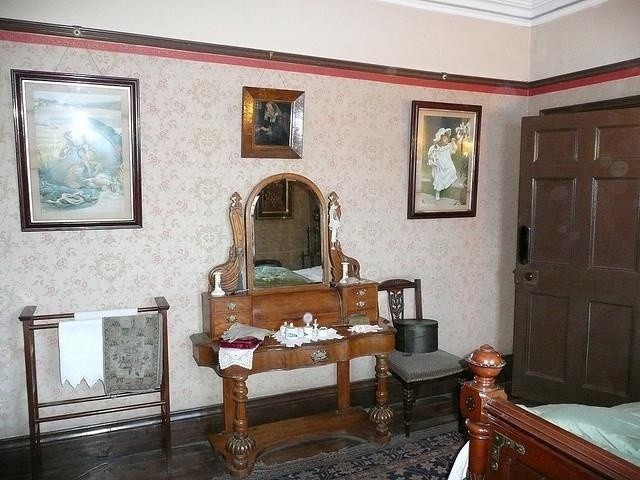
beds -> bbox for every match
[445,345,640,478]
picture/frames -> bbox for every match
[241,87,305,159]
[407,99,482,219]
[10,68,143,231]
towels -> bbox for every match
[57,306,164,398]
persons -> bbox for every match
[254,102,288,145]
[426,127,458,200]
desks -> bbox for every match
[191,276,398,477]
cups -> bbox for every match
[280,321,299,339]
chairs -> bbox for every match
[376,278,469,437]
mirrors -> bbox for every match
[245,173,330,295]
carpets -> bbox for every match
[211,419,469,480]
